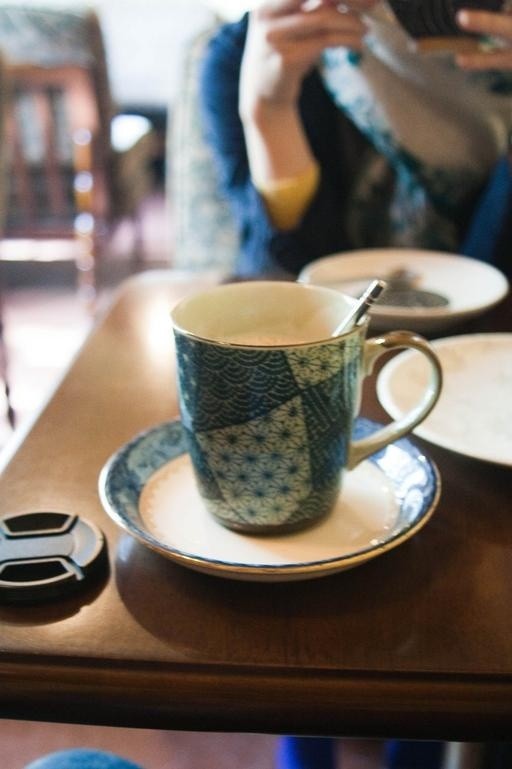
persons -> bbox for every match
[198,1,511,306]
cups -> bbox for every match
[170,279,443,538]
[346,0,508,53]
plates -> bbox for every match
[297,248,510,329]
[97,414,442,582]
[375,332,511,469]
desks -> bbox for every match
[0,264,511,767]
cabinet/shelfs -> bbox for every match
[2,0,109,295]
